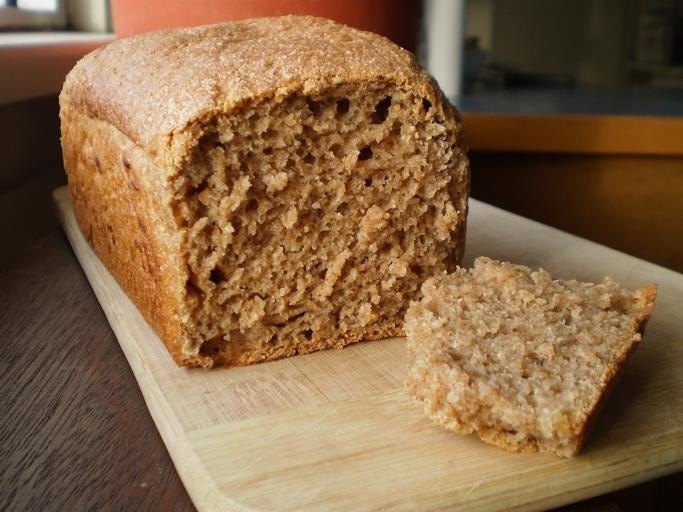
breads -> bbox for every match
[400,255,657,457]
[58,14,469,366]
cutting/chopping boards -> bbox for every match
[50,177,681,510]
